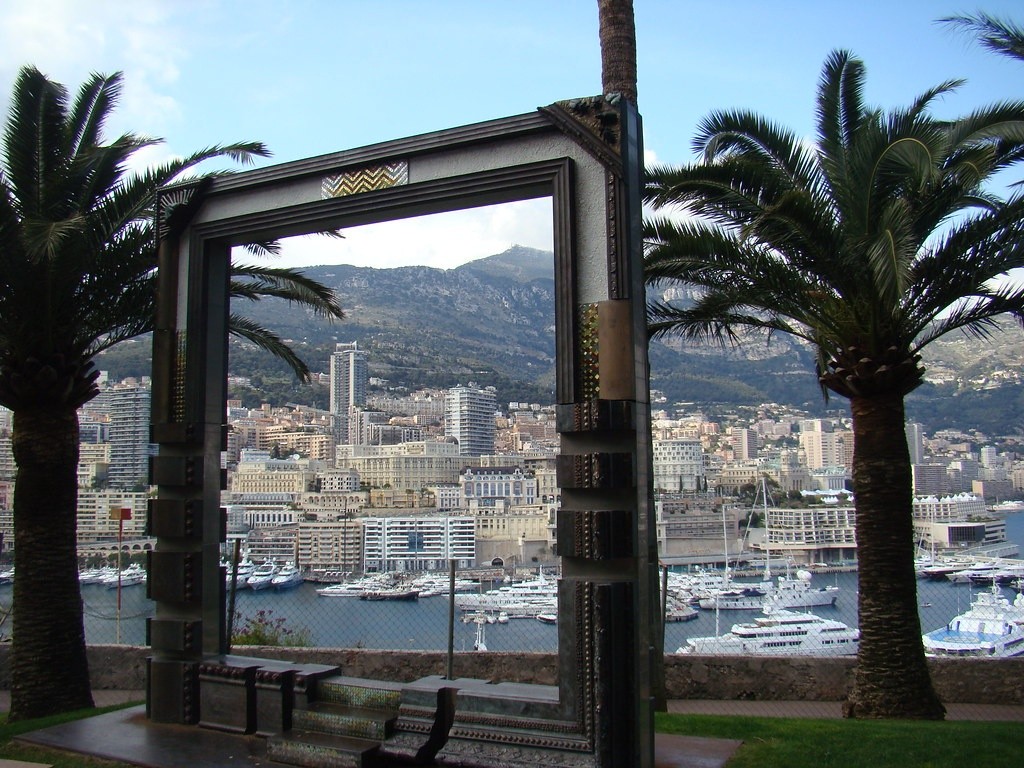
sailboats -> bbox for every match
[701,478,840,609]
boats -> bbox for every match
[675,609,859,656]
[1,562,308,592]
[917,550,1024,660]
[314,569,484,600]
[461,610,556,624]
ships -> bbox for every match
[442,574,698,622]
